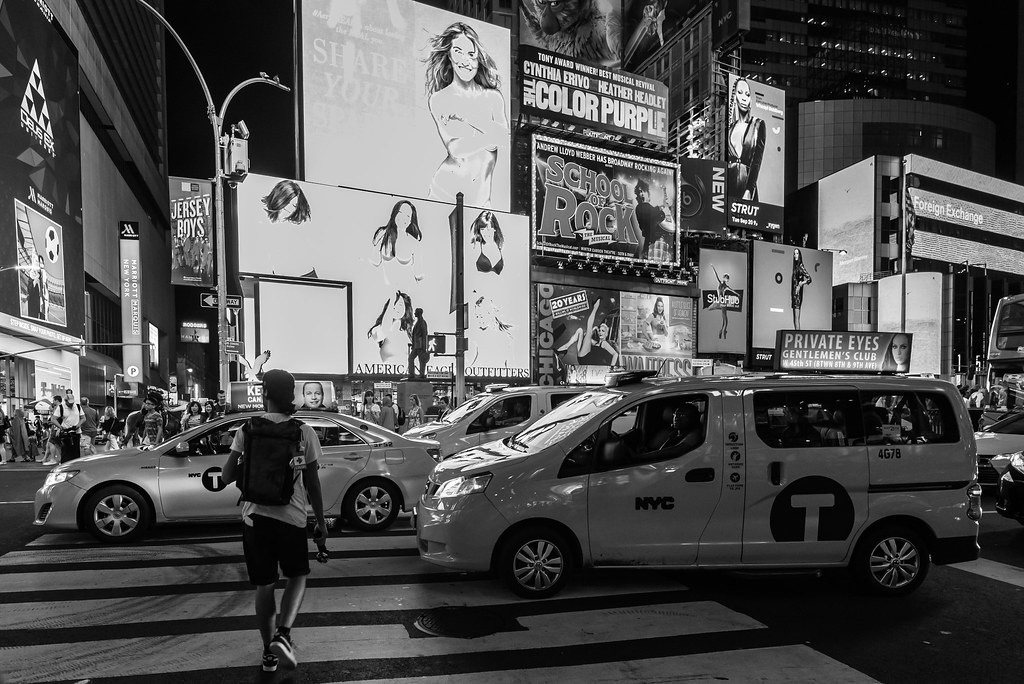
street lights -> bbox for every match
[113,372,126,418]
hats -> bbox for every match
[144,397,159,404]
[255,369,295,397]
[65,394,75,404]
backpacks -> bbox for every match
[392,403,406,425]
[234,416,305,507]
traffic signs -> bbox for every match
[200,291,247,313]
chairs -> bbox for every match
[644,403,680,452]
[813,407,848,447]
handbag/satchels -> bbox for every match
[49,424,63,444]
[3,416,11,430]
[101,431,111,443]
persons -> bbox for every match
[781,402,822,449]
[791,248,813,331]
[709,265,740,339]
[259,21,676,384]
[27,255,51,323]
[501,398,529,425]
[881,334,912,372]
[406,307,430,379]
[0,389,230,467]
[625,402,704,460]
[220,369,328,672]
[300,382,326,408]
[727,77,766,202]
[360,391,451,432]
[172,234,213,277]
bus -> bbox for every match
[984,295,1024,404]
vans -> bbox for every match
[411,331,985,601]
[402,382,607,462]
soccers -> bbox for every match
[45,225,60,263]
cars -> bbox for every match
[962,407,1024,525]
[32,409,444,547]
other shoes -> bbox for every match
[43,461,59,465]
[261,650,279,671]
[22,457,30,462]
[270,632,298,670]
[6,459,16,462]
